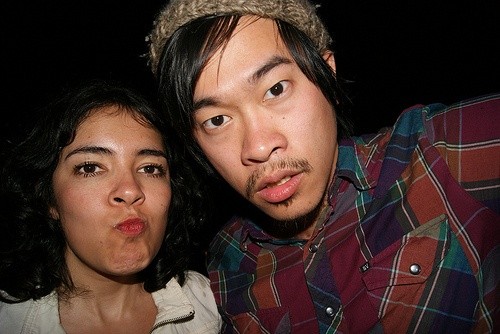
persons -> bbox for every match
[0,79,223,334]
[142,0,500,332]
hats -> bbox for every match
[145,0,331,73]
